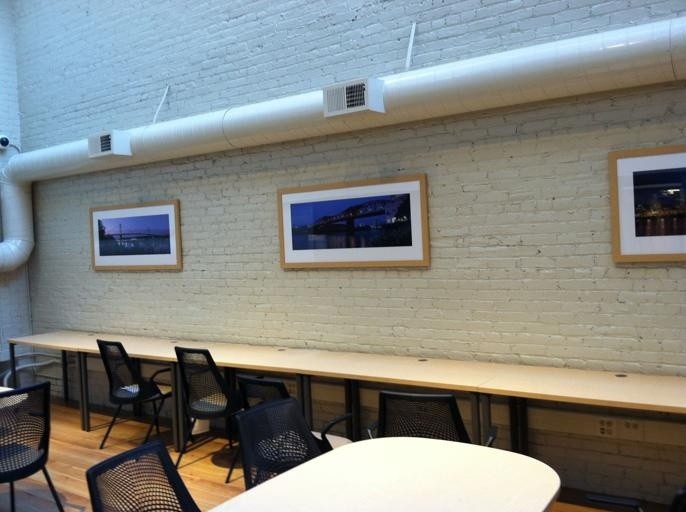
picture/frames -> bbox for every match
[277,173,431,270]
[607,144,686,264]
[88,200,184,272]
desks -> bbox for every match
[303,351,500,445]
[7,330,99,429]
[201,436,562,511]
[78,335,198,453]
[178,347,328,453]
[0,386,14,441]
[477,364,686,450]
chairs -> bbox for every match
[173,346,265,484]
[98,338,174,461]
[234,396,321,492]
[236,373,327,455]
[367,392,496,449]
[85,441,203,512]
[0,379,66,512]
